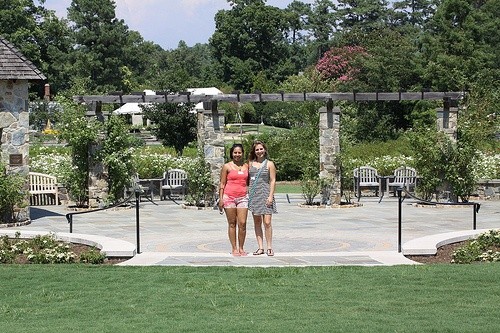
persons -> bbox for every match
[218,144,249,256]
[247,140,278,256]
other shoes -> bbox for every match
[238,251,247,256]
[232,251,242,256]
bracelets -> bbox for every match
[219,188,224,191]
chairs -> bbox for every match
[386,166,417,198]
[354,165,383,203]
[162,170,188,201]
[125,172,154,203]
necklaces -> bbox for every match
[238,168,241,171]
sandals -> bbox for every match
[253,249,264,255]
[267,249,275,256]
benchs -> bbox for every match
[29,172,59,206]
